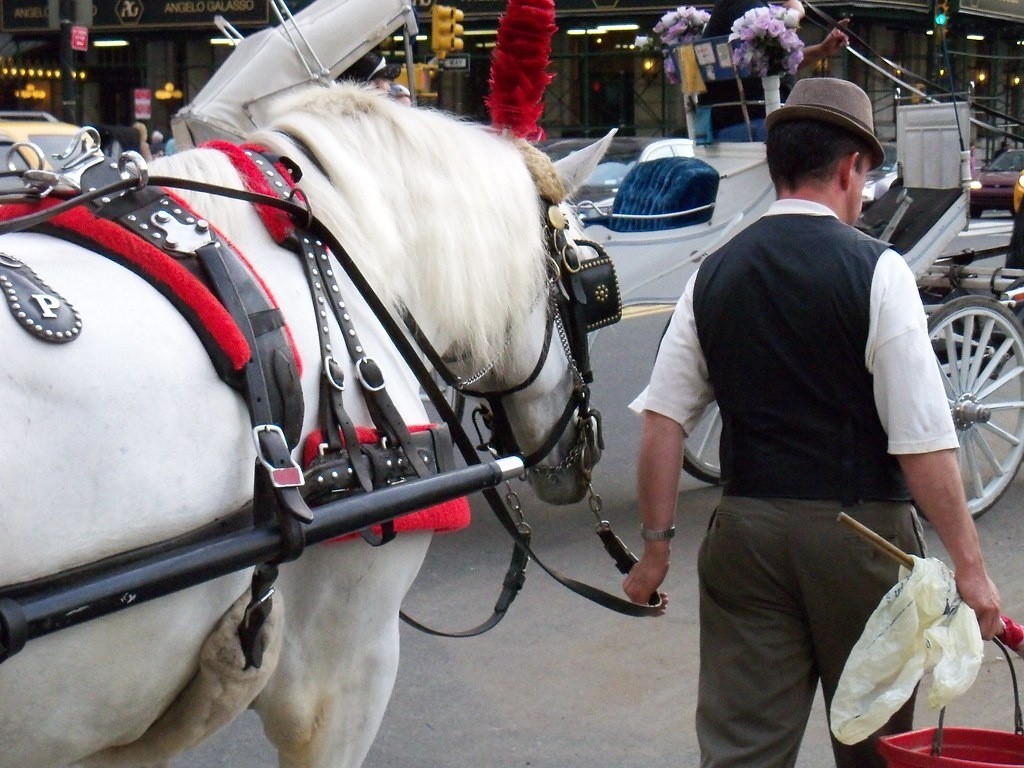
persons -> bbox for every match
[131,121,178,162]
[701,0,849,120]
[340,52,411,106]
[622,77,1001,768]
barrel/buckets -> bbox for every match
[878,633,1024,768]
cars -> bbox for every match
[541,136,671,217]
[970,149,1024,218]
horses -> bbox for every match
[0,82,618,768]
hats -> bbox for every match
[763,77,885,171]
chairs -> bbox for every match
[579,157,719,272]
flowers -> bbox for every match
[730,6,804,78]
[652,7,710,48]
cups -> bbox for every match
[75,0,97,26]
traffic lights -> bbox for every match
[934,13,948,28]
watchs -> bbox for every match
[640,524,676,541]
